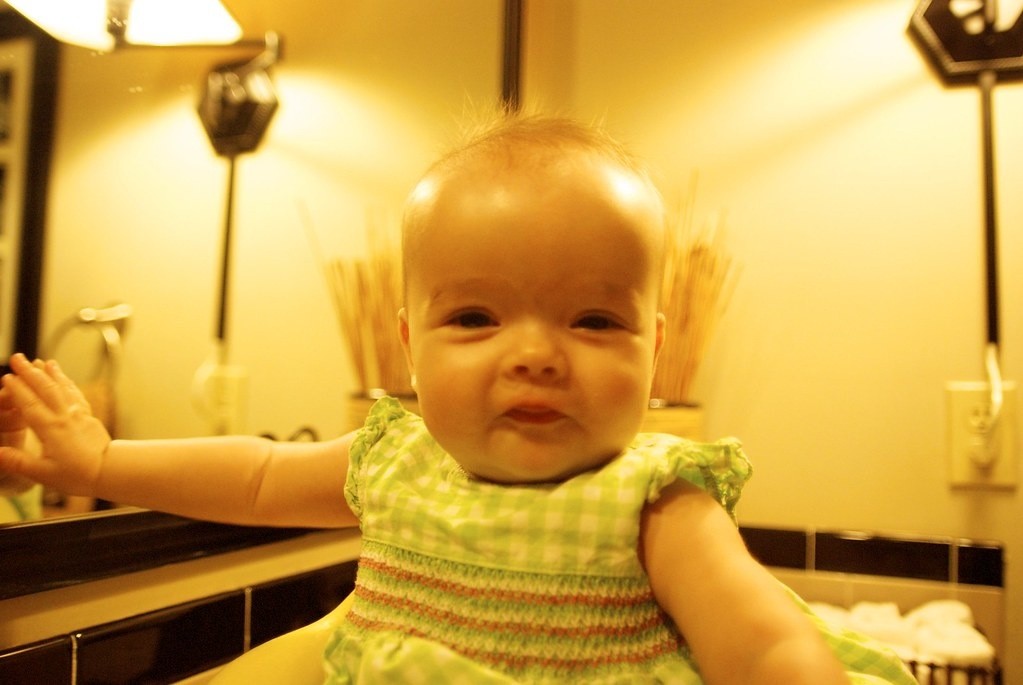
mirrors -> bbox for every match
[0,0,524,598]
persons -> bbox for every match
[0,117,919,685]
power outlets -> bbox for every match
[952,381,1016,485]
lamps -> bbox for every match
[3,0,243,52]
